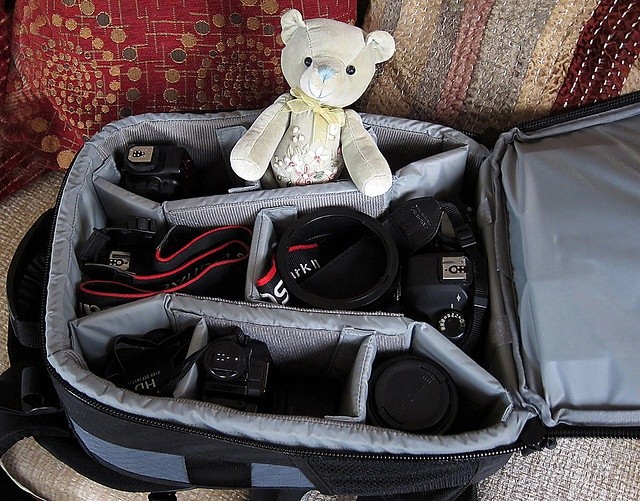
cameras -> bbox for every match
[276,207,474,341]
[120,144,191,199]
[196,340,270,410]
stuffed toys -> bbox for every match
[231,10,395,199]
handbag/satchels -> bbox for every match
[0,90,640,500]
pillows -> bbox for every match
[355,1,639,144]
[1,0,359,169]
